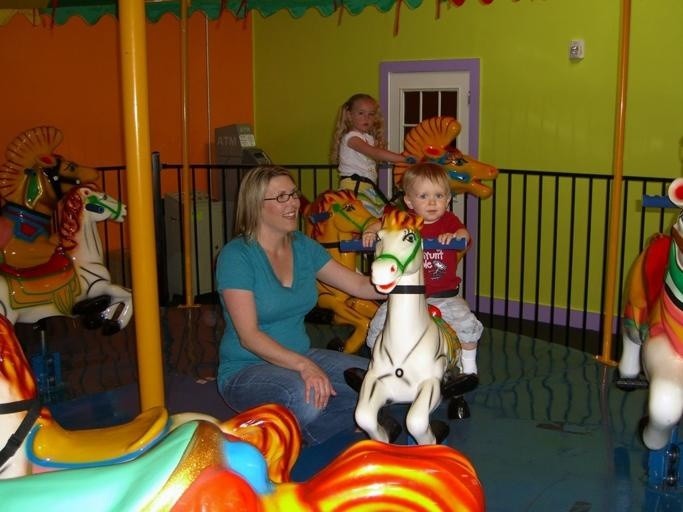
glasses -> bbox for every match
[263,189,301,203]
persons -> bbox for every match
[331,94,408,218]
[362,164,484,377]
[216,165,388,450]
